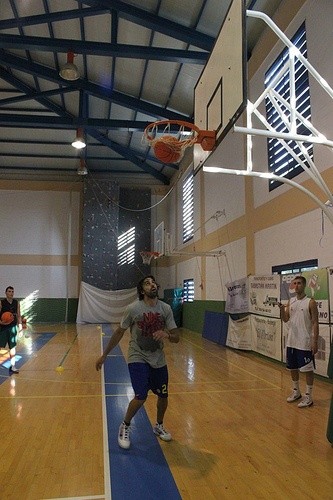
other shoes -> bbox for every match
[9,367,19,373]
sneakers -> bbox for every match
[118,421,130,449]
[153,423,172,441]
[287,394,301,402]
[298,394,312,407]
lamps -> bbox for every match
[71,136,86,149]
[59,52,80,81]
[77,160,88,175]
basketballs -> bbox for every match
[154,136,180,163]
[1,312,13,322]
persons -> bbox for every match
[277,276,318,407]
[96,275,180,450]
[0,285,21,373]
[326,342,333,447]
